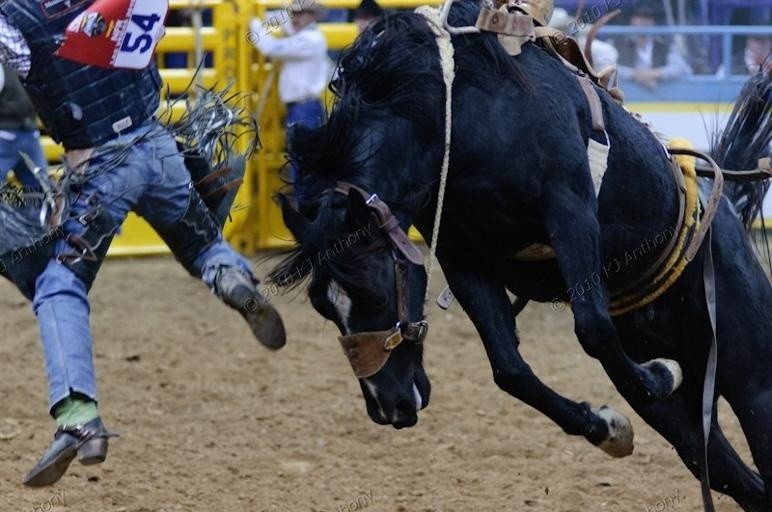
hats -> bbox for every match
[285,1,326,21]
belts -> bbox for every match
[288,97,319,107]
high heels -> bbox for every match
[22,396,110,487]
[213,264,287,351]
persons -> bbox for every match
[711,22,772,75]
[352,1,385,32]
[551,8,618,86]
[248,1,335,198]
[603,4,686,91]
[0,0,289,487]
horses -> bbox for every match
[250,10,771,511]
